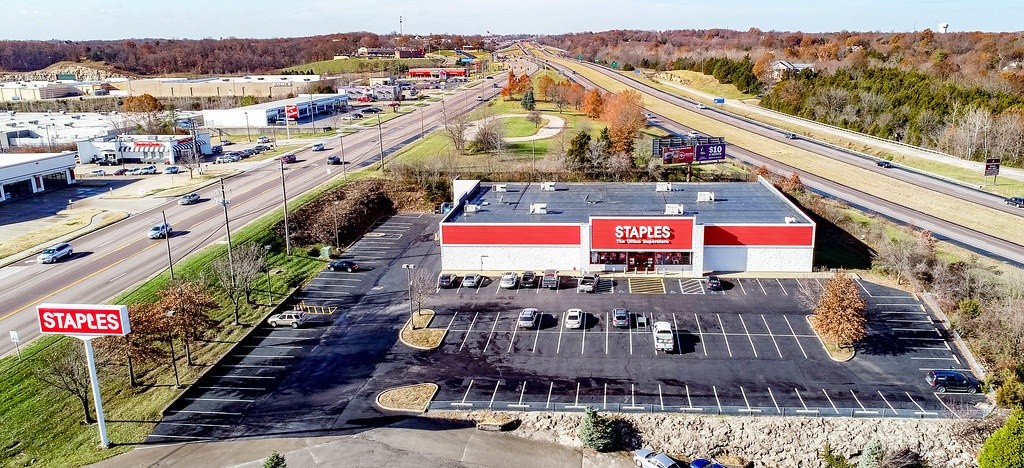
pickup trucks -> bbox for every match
[578,272,601,292]
[520,270,537,288]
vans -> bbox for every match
[652,321,675,354]
[540,269,561,289]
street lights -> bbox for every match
[401,262,417,331]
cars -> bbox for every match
[646,113,653,119]
[688,129,700,138]
[313,143,325,151]
[178,193,201,204]
[925,368,982,395]
[611,307,629,327]
[90,164,180,175]
[327,156,342,165]
[565,308,583,329]
[783,132,797,140]
[499,271,519,288]
[633,449,681,468]
[213,136,274,164]
[438,273,456,288]
[697,103,706,109]
[876,160,891,168]
[517,307,539,328]
[461,273,481,288]
[688,458,725,468]
[37,243,74,265]
[148,222,172,239]
[328,261,361,273]
[341,107,383,119]
[388,101,400,106]
[1004,195,1024,208]
[281,154,296,164]
[703,273,723,291]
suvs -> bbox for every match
[267,311,308,327]
